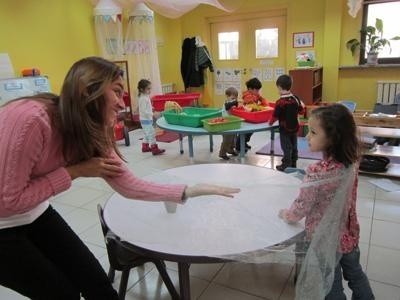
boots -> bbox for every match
[151,144,166,155]
[142,143,152,152]
[292,158,296,168]
[276,159,292,171]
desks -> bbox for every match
[104,163,305,296]
[155,108,280,161]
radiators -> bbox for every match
[376,81,400,114]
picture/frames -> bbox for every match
[291,30,314,49]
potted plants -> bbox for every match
[345,18,399,64]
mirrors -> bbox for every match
[110,59,134,125]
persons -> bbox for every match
[236,78,263,153]
[219,86,238,160]
[268,74,305,171]
[0,57,240,300]
[278,104,376,300]
[137,79,166,157]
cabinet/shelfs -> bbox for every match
[288,66,326,106]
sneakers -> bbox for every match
[245,142,251,148]
[219,153,231,160]
[228,150,239,156]
[236,147,248,153]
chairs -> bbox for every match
[96,204,178,296]
